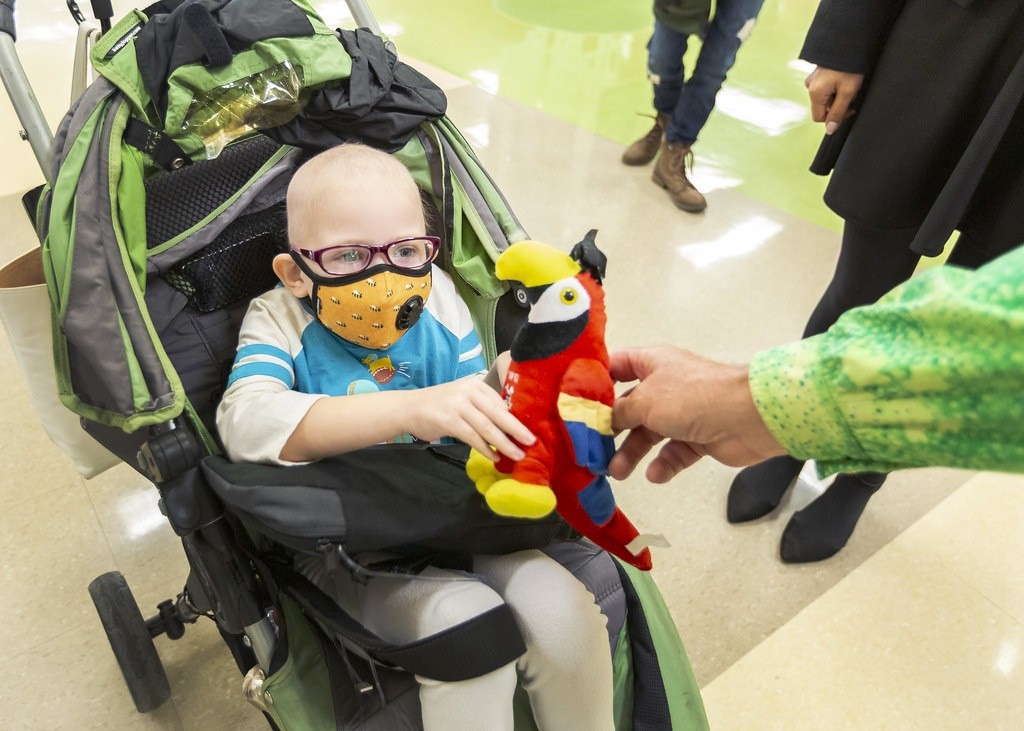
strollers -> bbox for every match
[1,1,713,731]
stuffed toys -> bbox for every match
[466,241,658,573]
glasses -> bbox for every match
[288,235,441,276]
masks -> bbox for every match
[288,250,432,351]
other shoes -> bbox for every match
[727,485,775,524]
[779,511,842,563]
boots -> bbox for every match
[651,138,707,212]
[620,113,672,165]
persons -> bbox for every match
[622,0,766,213]
[725,0,1023,569]
[213,143,618,731]
[603,238,1023,486]
